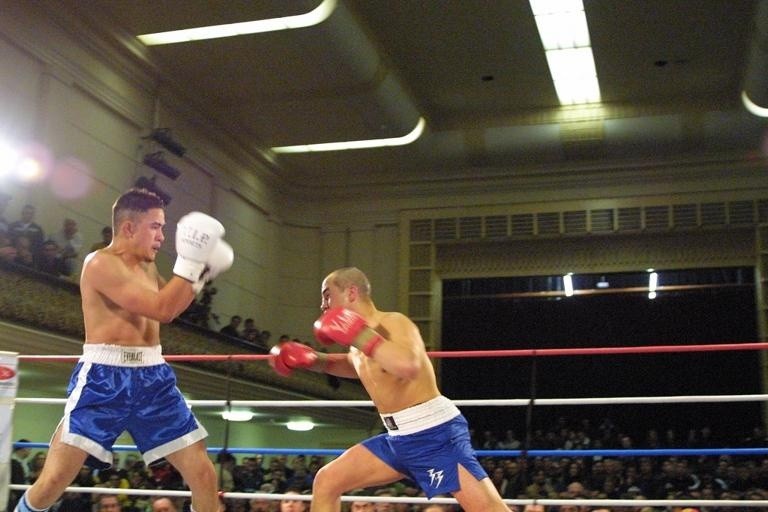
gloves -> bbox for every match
[268,340,328,379]
[312,304,383,357]
[172,211,234,294]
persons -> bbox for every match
[213,449,465,512]
[6,439,192,512]
[2,205,113,284]
[264,264,512,510]
[179,280,328,353]
[12,188,238,511]
[469,415,766,510]
